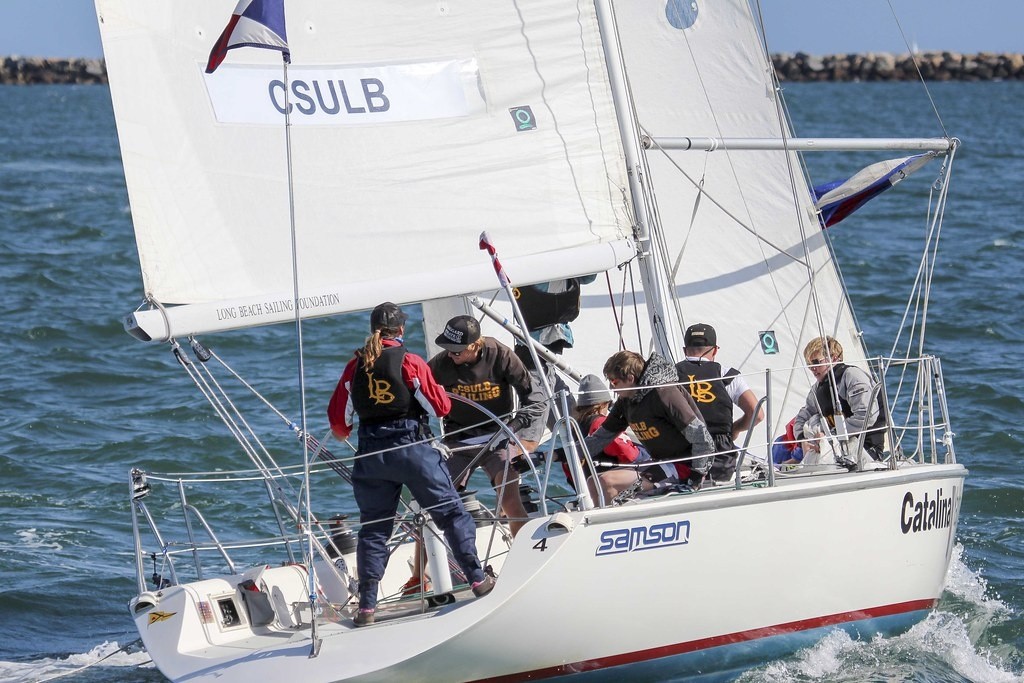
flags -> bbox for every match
[205,0,290,74]
[810,151,933,232]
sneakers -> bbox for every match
[469,571,499,597]
[352,608,375,626]
[399,576,430,594]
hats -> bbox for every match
[577,374,613,406]
[684,323,716,346]
[370,302,409,332]
[435,315,480,353]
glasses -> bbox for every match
[806,357,825,370]
[451,352,460,356]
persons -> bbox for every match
[402,315,546,595]
[327,302,498,627]
[793,335,887,460]
[513,273,598,454]
[561,374,639,493]
[673,323,765,482]
[510,351,715,508]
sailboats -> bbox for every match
[91,0,968,683]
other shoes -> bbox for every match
[523,501,538,513]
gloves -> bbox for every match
[510,452,546,475]
[678,470,706,494]
